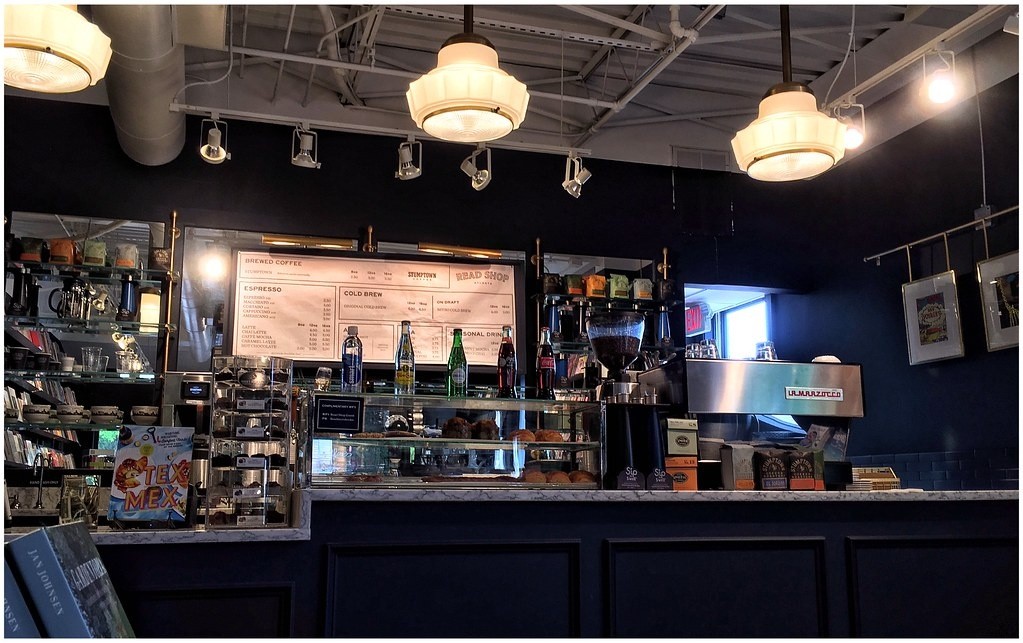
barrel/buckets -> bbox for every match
[613,383,640,404]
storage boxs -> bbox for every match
[719,444,827,491]
[663,417,699,490]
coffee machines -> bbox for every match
[583,304,648,401]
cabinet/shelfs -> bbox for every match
[306,391,606,489]
[531,238,679,400]
[4,207,295,531]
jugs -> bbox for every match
[49,279,91,327]
[189,459,209,489]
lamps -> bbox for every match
[923,49,955,101]
[292,121,321,169]
[394,5,530,190]
[200,114,231,164]
[4,5,114,94]
[685,302,715,338]
[730,5,865,182]
[562,150,591,199]
[1003,11,1019,36]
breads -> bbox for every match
[441,418,500,440]
[503,428,595,484]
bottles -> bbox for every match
[497,326,518,399]
[389,469,398,488]
[555,353,568,389]
[603,302,671,346]
[535,327,552,400]
[395,321,415,399]
[10,495,21,509]
[586,363,598,390]
[448,329,468,400]
[341,326,363,394]
[546,298,595,343]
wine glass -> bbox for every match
[312,366,333,394]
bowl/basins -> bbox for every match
[131,406,159,425]
[57,405,124,425]
[4,405,59,424]
[390,459,400,463]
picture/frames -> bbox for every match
[902,270,964,365]
[977,249,1019,352]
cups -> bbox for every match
[91,356,109,382]
[606,393,657,405]
[61,357,74,371]
[81,347,102,371]
[73,365,81,377]
[685,339,718,359]
[743,342,778,361]
[116,351,144,373]
